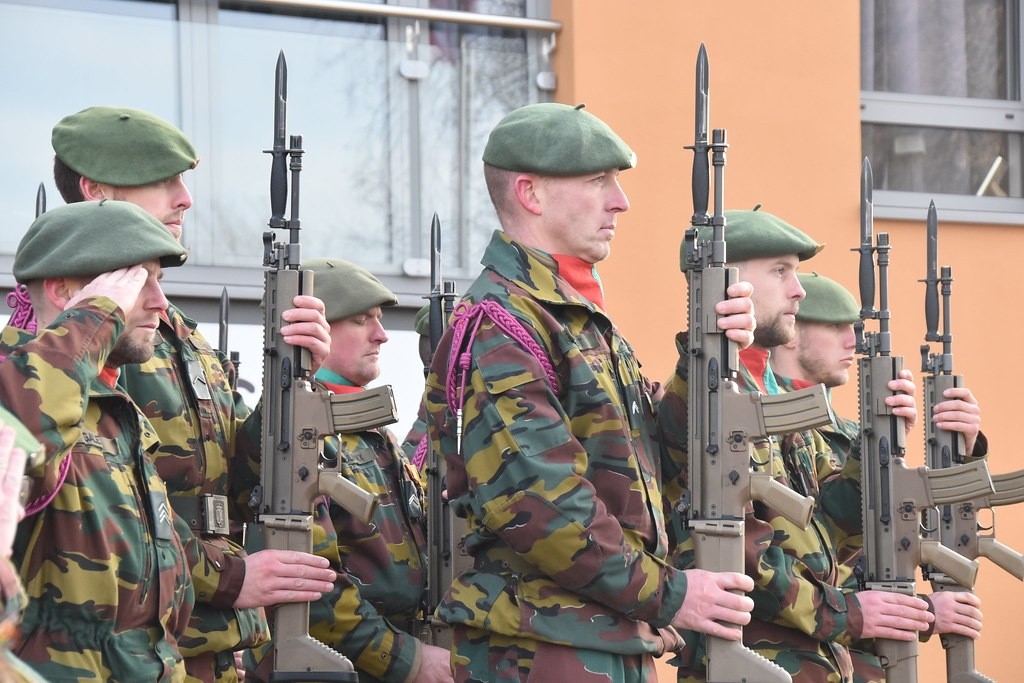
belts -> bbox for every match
[169,494,248,537]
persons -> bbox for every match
[234,254,455,683]
[0,100,342,683]
[402,103,755,683]
[656,209,990,683]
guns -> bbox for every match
[851,155,998,683]
[678,42,835,683]
[416,212,461,653]
[922,198,1024,683]
[217,286,243,389]
[249,46,400,682]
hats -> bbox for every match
[679,203,825,273]
[299,257,399,323]
[414,299,462,337]
[483,102,638,176]
[798,271,864,324]
[51,106,200,186]
[12,198,191,283]
